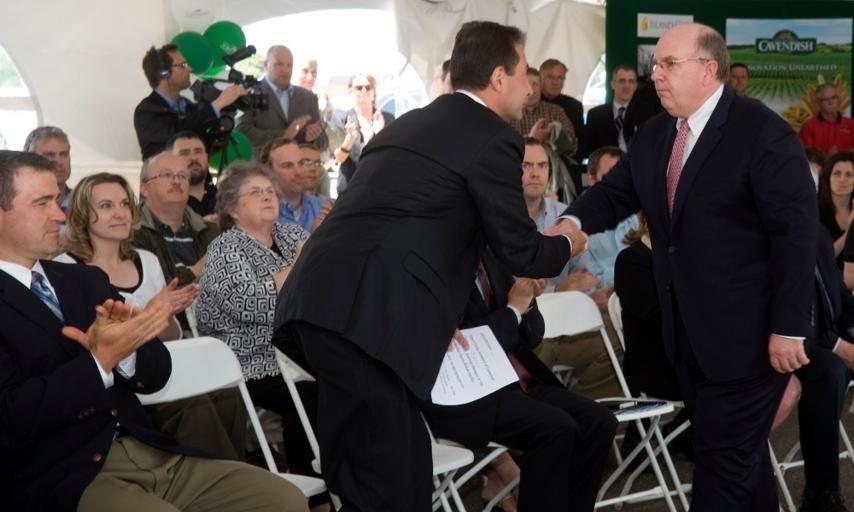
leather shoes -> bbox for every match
[800,486,848,512]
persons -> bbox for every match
[511,57,664,399]
[330,68,397,196]
[270,21,587,512]
[0,128,336,512]
[134,44,249,161]
[771,82,854,512]
[730,62,750,94]
[231,44,335,161]
[419,245,618,511]
[544,24,820,510]
[613,213,803,510]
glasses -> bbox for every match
[144,169,189,184]
[651,56,710,72]
[355,85,372,90]
[817,95,838,102]
[170,61,188,70]
[302,158,325,167]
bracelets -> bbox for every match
[339,146,353,155]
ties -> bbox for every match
[476,260,533,396]
[666,118,689,222]
[30,271,65,327]
[614,107,625,138]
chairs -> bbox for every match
[275,346,475,511]
[765,417,853,512]
[180,285,202,339]
[595,293,695,512]
[132,337,328,511]
[534,292,679,510]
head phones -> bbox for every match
[156,50,171,78]
